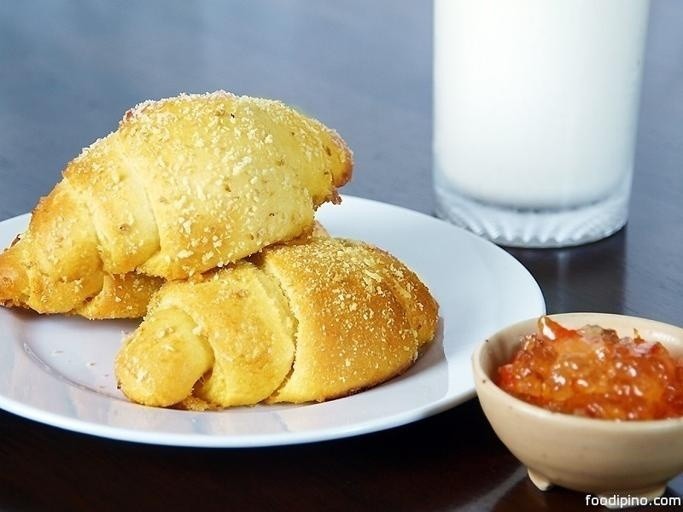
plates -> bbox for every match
[0,195,546,448]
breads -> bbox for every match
[20,93,354,284]
[1,220,331,321]
[116,237,441,413]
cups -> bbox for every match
[433,0,651,249]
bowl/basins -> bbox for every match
[473,312,683,510]
